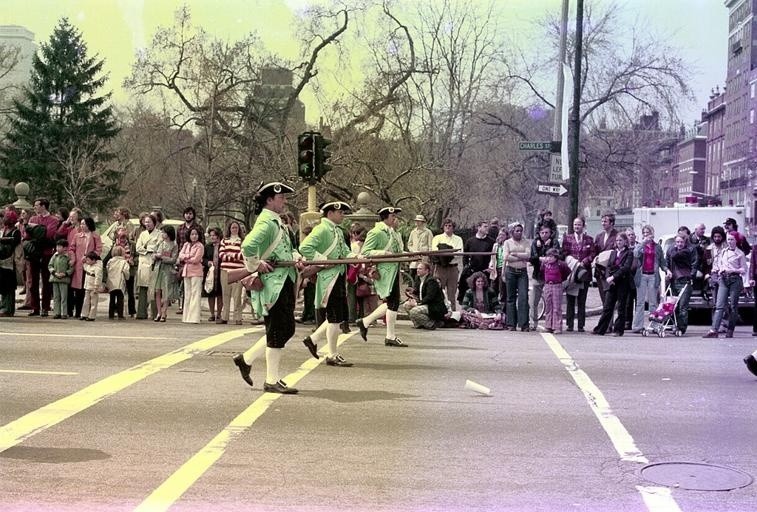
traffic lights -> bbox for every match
[312,133,334,181]
[297,131,315,181]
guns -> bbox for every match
[226,256,421,285]
[375,250,499,257]
[302,248,460,277]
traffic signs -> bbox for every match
[548,153,566,184]
[536,183,570,198]
[517,140,553,151]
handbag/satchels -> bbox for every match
[204,266,215,294]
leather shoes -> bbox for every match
[265,379,298,394]
[356,319,368,342]
[385,336,408,347]
[326,356,354,366]
[302,336,319,359]
[234,353,253,386]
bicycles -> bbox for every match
[398,267,416,305]
[516,288,551,321]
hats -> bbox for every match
[377,207,401,215]
[414,215,427,222]
[255,183,294,198]
[574,266,587,284]
[84,251,101,260]
[319,202,352,213]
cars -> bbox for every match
[95,218,185,259]
[556,224,569,233]
[655,234,756,311]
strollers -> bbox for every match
[641,276,695,338]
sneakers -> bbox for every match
[251,319,265,325]
[208,315,243,325]
[591,324,632,336]
[566,326,574,331]
[109,310,167,323]
[703,329,718,338]
[578,328,584,332]
[506,327,530,331]
[544,328,562,334]
[726,330,733,338]
[16,304,96,321]
[176,310,182,314]
[295,317,315,324]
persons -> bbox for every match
[0,198,248,325]
[403,215,756,375]
[233,182,422,392]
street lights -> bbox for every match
[191,176,197,207]
[687,170,722,205]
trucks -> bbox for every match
[633,205,748,248]
